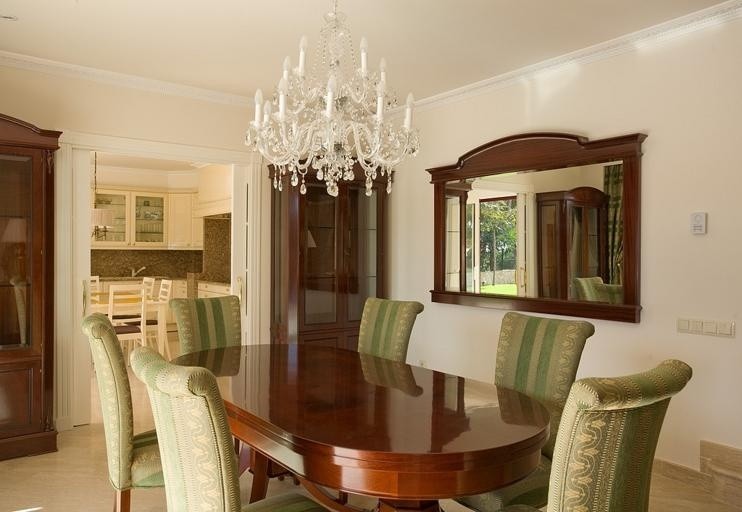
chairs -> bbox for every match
[81,312,239,512]
[358,296,425,363]
[130,346,330,512]
[124,279,172,361]
[108,285,147,368]
[448,312,595,512]
[90,275,108,317]
[596,283,625,305]
[111,276,156,353]
[169,295,241,355]
[492,358,693,512]
[573,277,604,302]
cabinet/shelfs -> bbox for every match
[89,282,103,292]
[268,143,394,351]
[143,280,173,332]
[0,111,62,463]
[91,182,168,250]
[173,280,187,331]
[104,281,142,294]
[168,186,199,250]
[534,187,609,301]
[198,282,231,299]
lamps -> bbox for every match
[91,209,114,240]
[244,0,421,197]
[2,216,30,286]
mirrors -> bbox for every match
[425,133,650,324]
